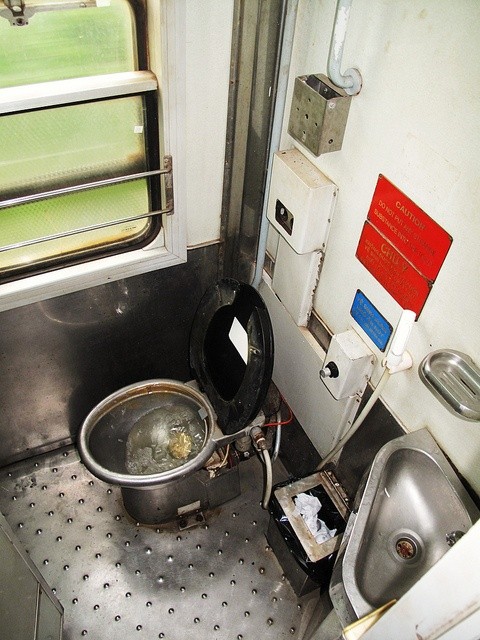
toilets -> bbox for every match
[77,281,275,524]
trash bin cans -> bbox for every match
[263,463,354,596]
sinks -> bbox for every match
[326,425,479,628]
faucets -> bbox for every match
[444,527,466,549]
